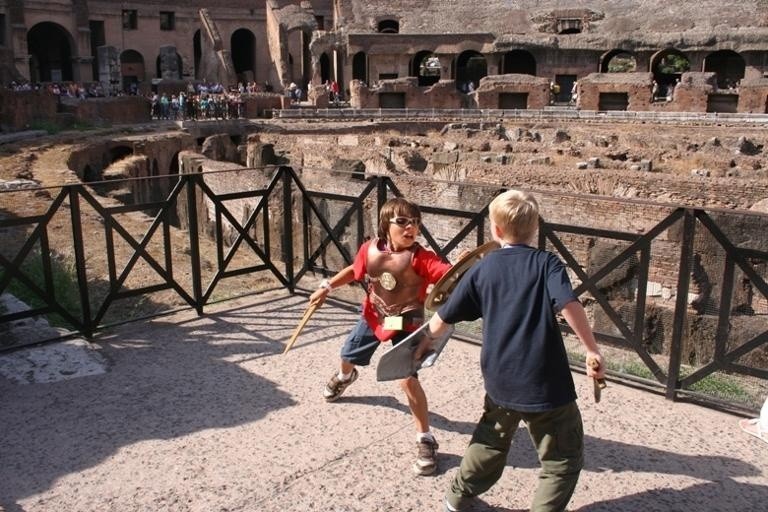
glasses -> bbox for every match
[389,216,422,227]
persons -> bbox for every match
[723,78,740,92]
[420,66,441,76]
[462,81,467,91]
[309,198,454,476]
[467,79,475,91]
[549,78,579,106]
[4,79,274,120]
[285,79,313,102]
[653,77,682,102]
[413,190,606,510]
[324,79,339,105]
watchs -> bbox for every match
[319,280,333,293]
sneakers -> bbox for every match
[323,368,359,402]
[446,494,489,512]
[413,435,439,476]
